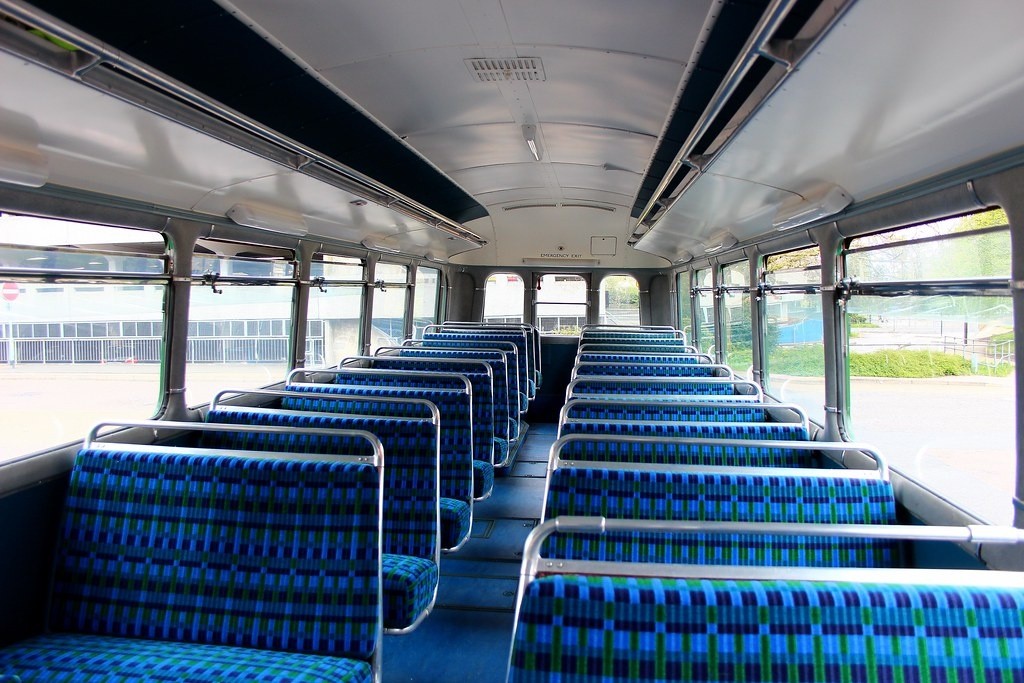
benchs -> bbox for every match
[0,321,1024,683]
